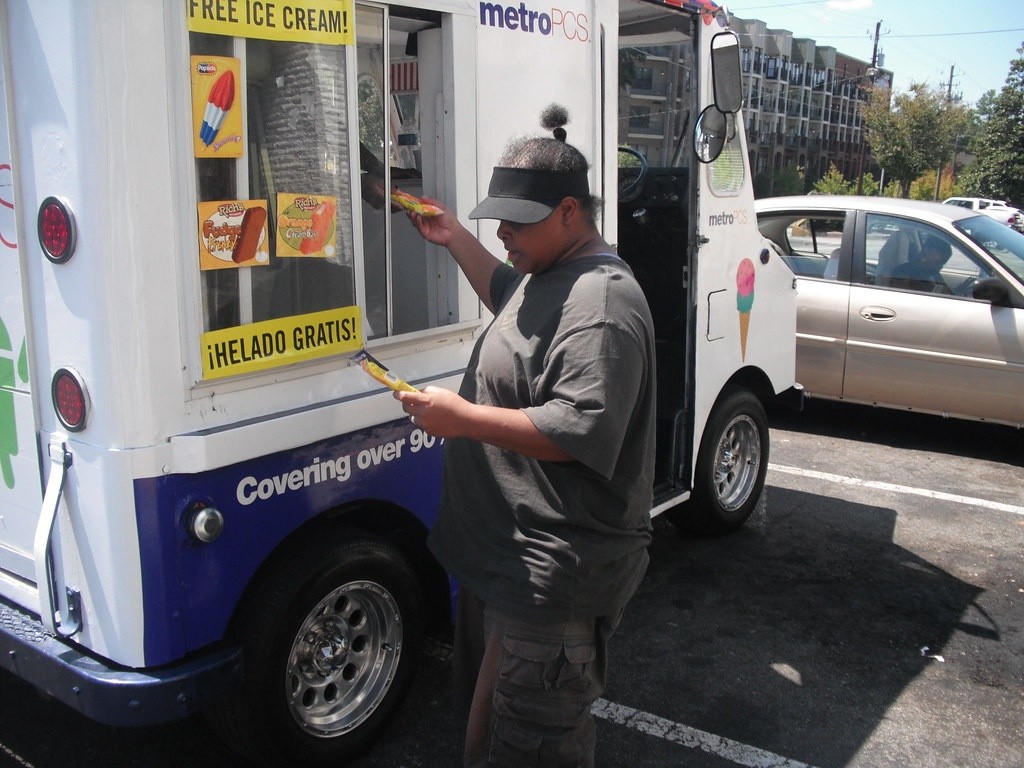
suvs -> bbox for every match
[940,195,1008,212]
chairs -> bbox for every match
[822,225,912,290]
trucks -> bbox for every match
[0,0,804,765]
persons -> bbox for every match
[891,234,956,296]
[394,133,665,768]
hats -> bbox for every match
[467,166,588,225]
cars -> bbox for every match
[750,195,1024,432]
